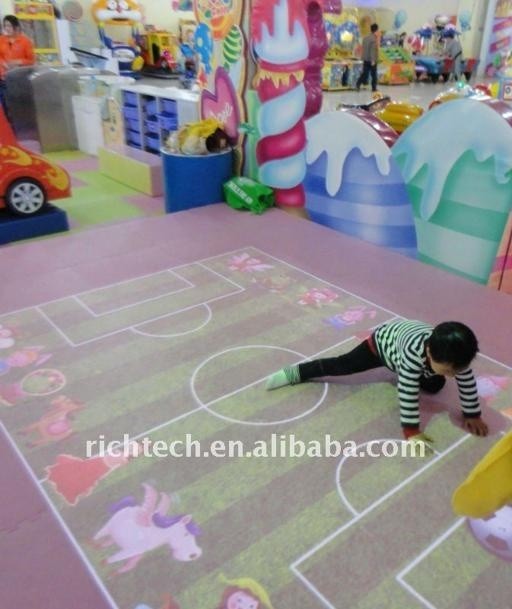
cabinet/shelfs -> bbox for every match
[70,71,201,159]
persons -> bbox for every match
[352,21,379,90]
[440,35,462,80]
[264,319,489,455]
[0,15,37,130]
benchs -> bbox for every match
[99,145,163,200]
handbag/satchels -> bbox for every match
[223,176,276,215]
